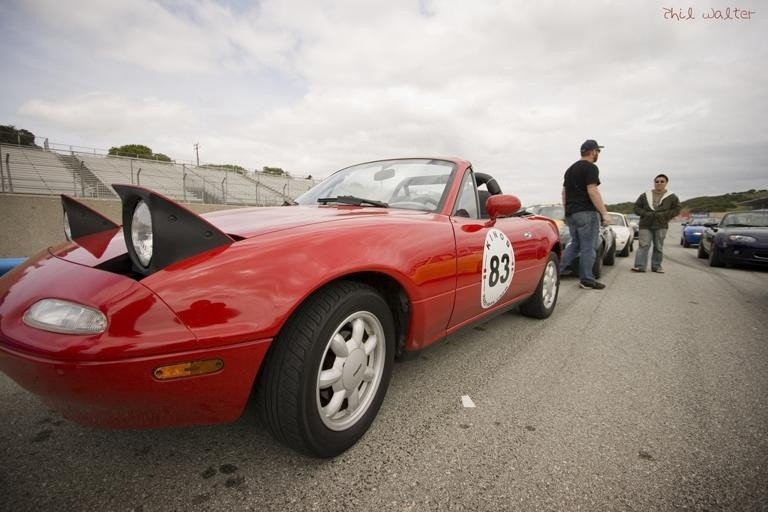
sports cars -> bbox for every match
[2,158,562,459]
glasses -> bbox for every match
[595,150,602,153]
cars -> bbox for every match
[523,203,642,266]
[680,210,768,267]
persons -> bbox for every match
[630,173,680,273]
[558,140,613,289]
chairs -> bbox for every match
[460,190,492,220]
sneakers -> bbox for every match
[630,266,665,273]
[577,279,606,289]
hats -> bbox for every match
[580,139,605,150]
